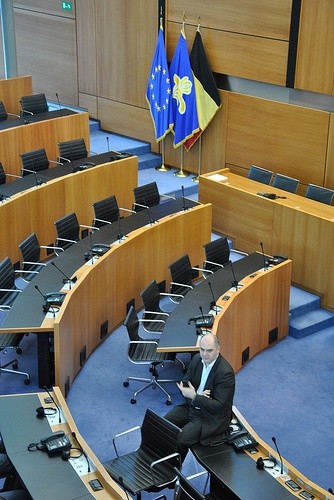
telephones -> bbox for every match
[45,292,66,305]
[116,152,132,159]
[224,431,259,451]
[77,161,95,170]
[40,430,72,454]
[91,244,111,255]
[194,314,215,328]
[267,254,288,265]
[257,192,276,200]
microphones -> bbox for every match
[119,476,129,500]
[229,260,238,291]
[328,488,334,495]
[207,281,217,316]
[198,306,208,334]
[71,432,90,472]
[2,91,190,315]
[272,437,283,474]
[259,242,265,271]
[43,386,61,423]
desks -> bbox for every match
[0,385,134,500]
[156,250,292,372]
[199,168,334,313]
[190,402,334,500]
[0,108,90,182]
[0,150,138,273]
[0,196,213,401]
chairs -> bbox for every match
[0,94,334,500]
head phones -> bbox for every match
[62,447,82,461]
[61,277,75,284]
[209,301,222,312]
[196,328,211,336]
[232,281,243,289]
[35,407,58,418]
[224,423,242,435]
[42,305,60,313]
[256,457,276,470]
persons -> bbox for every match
[164,333,235,464]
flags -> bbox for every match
[145,25,174,143]
[168,30,200,149]
[183,31,223,152]
